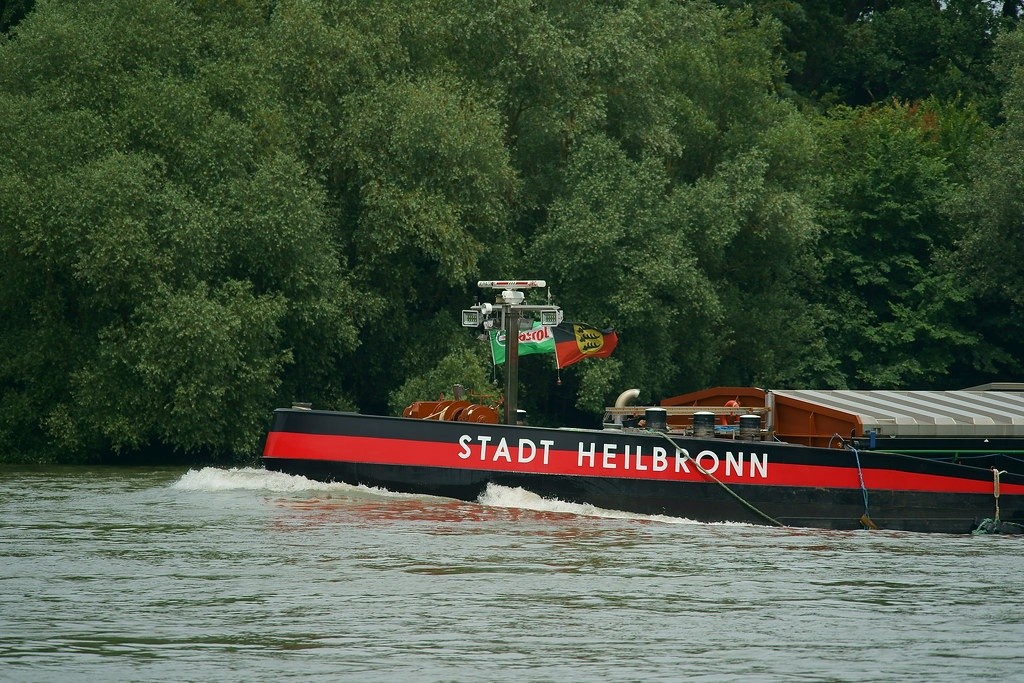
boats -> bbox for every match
[254,281,1024,533]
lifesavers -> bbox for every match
[721,400,740,425]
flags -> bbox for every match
[489,321,556,365]
[550,321,618,370]
